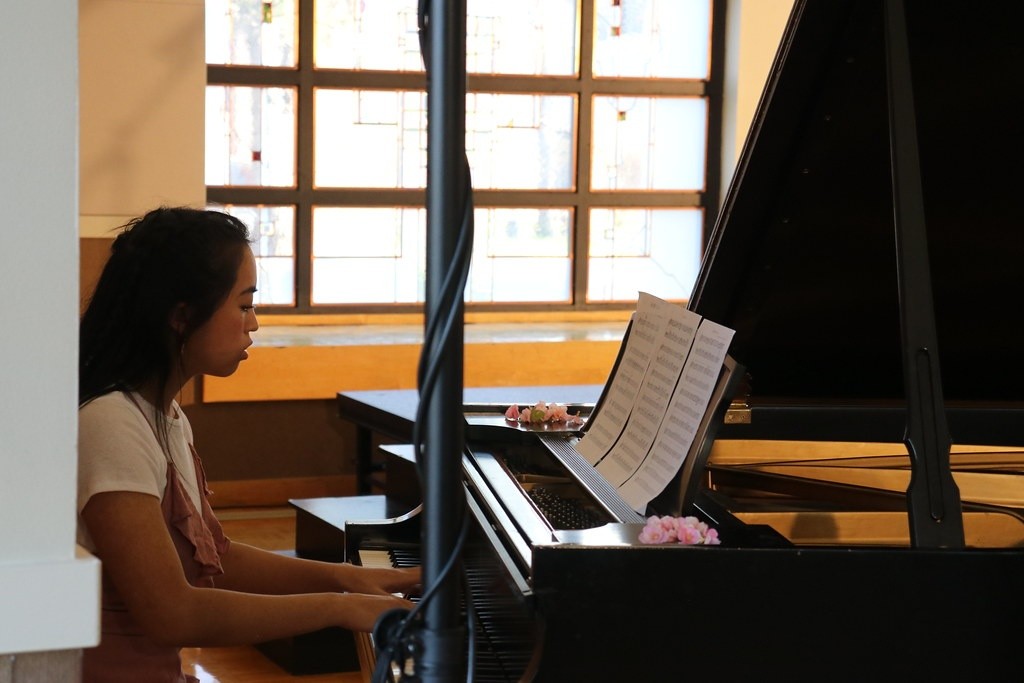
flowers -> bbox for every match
[506,405,581,427]
[640,514,721,547]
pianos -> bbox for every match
[343,1,1024,682]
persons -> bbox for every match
[80,206,422,683]
